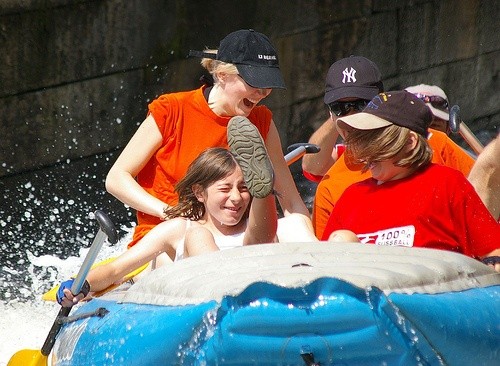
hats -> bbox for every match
[324,55,384,104]
[190,29,287,90]
[404,84,449,120]
[335,90,433,142]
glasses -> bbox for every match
[413,93,449,111]
[328,100,371,116]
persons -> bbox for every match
[56,117,277,306]
[312,54,500,245]
[302,84,500,183]
[105,29,317,248]
[324,90,500,273]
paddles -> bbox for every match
[5,210,119,366]
[451,105,483,155]
[282,143,322,168]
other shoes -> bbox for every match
[227,116,275,199]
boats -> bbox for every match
[50,211,500,366]
